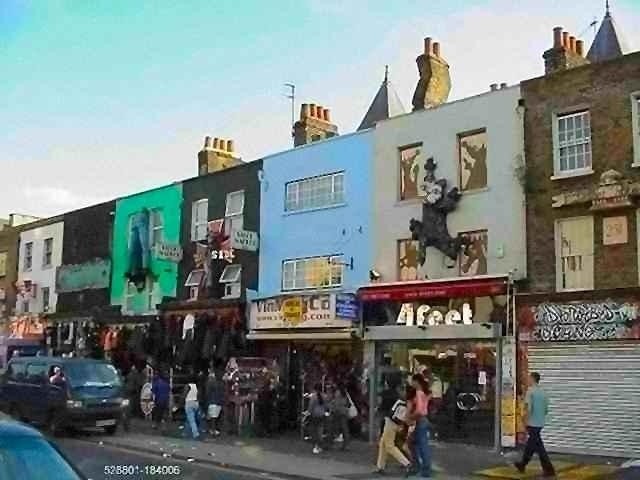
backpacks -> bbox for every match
[386,391,408,426]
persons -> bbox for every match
[49,355,559,480]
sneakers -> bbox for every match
[398,465,433,478]
[312,447,324,455]
[372,468,384,474]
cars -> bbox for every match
[0,409,95,480]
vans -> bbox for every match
[0,356,129,437]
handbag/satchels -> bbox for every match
[344,392,358,420]
